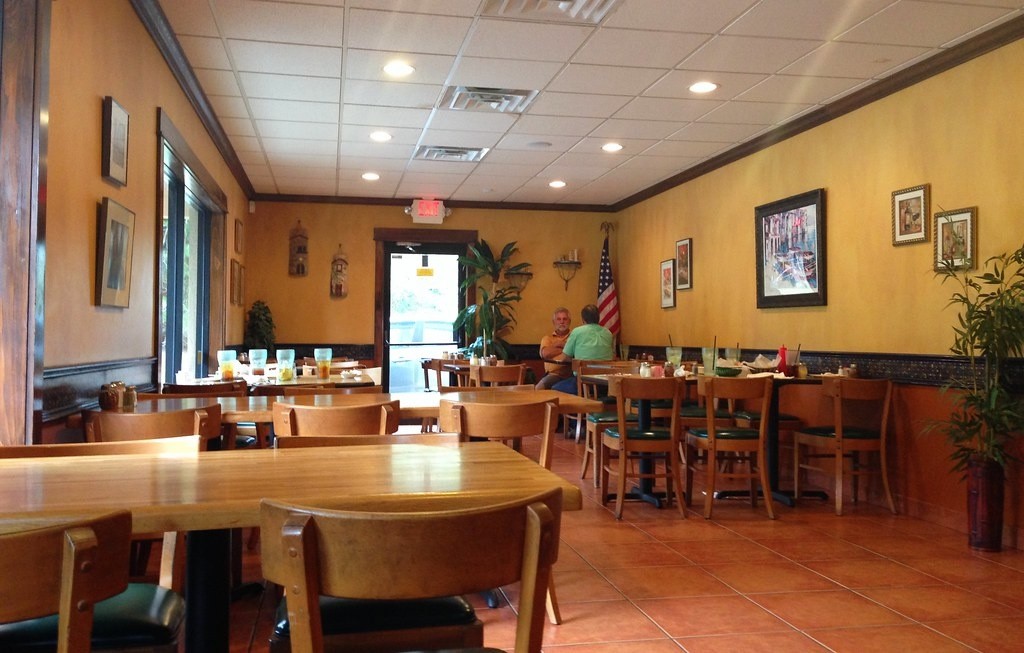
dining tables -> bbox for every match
[69,388,604,469]
[3,440,584,644]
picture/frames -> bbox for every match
[660,258,677,309]
[95,197,136,309]
[675,238,691,289]
[755,188,827,307]
[891,182,931,244]
[235,218,244,254]
[102,94,129,187]
[933,205,979,271]
[230,258,246,306]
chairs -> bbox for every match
[0,348,898,653]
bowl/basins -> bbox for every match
[339,373,357,378]
[716,365,742,377]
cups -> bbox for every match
[784,350,800,376]
[725,348,742,361]
[216,350,236,381]
[702,347,718,376]
[665,346,682,368]
[276,349,294,380]
[314,348,332,380]
[249,349,267,381]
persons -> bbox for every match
[535,306,574,389]
[552,304,614,439]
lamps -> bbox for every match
[404,199,452,225]
[504,268,533,302]
[553,260,582,292]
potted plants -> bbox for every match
[930,206,1024,551]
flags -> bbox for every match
[596,237,621,337]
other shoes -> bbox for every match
[569,426,584,438]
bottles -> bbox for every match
[797,362,807,377]
[238,352,247,362]
[443,351,464,359]
[664,361,674,377]
[469,354,497,366]
[99,380,138,409]
[849,362,858,378]
[779,344,787,374]
[838,366,844,374]
[640,362,652,379]
